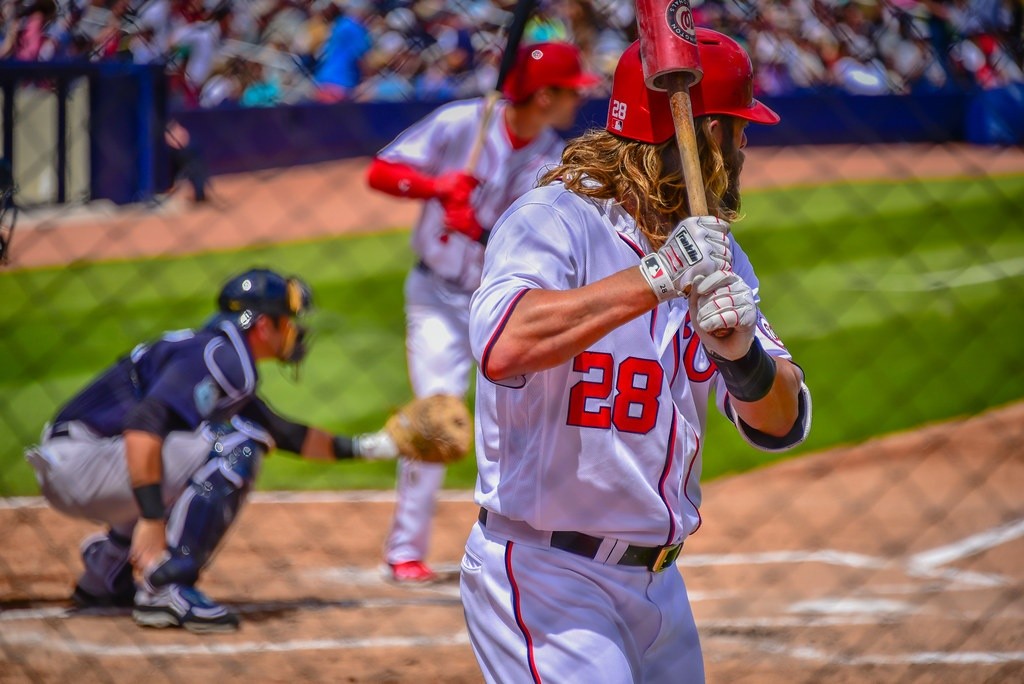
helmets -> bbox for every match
[503,43,599,100]
[604,27,781,144]
[218,267,310,318]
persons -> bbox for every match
[363,42,603,588]
[0,0,1024,108]
[26,265,473,635]
[459,25,814,683]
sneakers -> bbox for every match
[132,585,238,633]
[74,584,136,612]
[386,560,436,587]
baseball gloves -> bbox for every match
[383,392,473,464]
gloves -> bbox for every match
[689,270,755,360]
[639,215,733,303]
[443,200,482,241]
[432,173,479,205]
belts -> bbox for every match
[478,507,683,574]
[50,422,70,436]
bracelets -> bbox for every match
[133,484,166,521]
[702,334,778,402]
[334,436,353,461]
[477,228,490,246]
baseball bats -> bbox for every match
[634,1,734,342]
[439,2,540,246]
